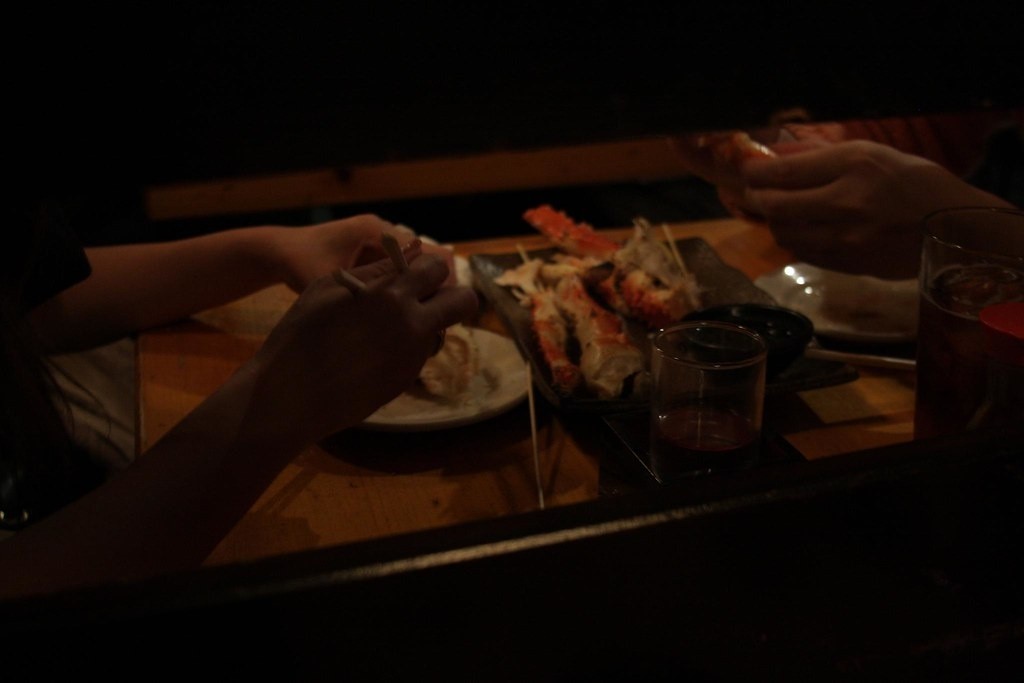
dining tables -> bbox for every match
[132,221,1024,621]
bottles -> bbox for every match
[978,302,1024,440]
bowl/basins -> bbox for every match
[676,304,814,382]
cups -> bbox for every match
[914,207,1024,455]
[648,325,768,487]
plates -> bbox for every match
[472,232,860,402]
[754,262,916,344]
[365,326,533,429]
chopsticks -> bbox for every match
[330,232,407,300]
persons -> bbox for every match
[0,196,478,633]
[668,123,1024,282]
